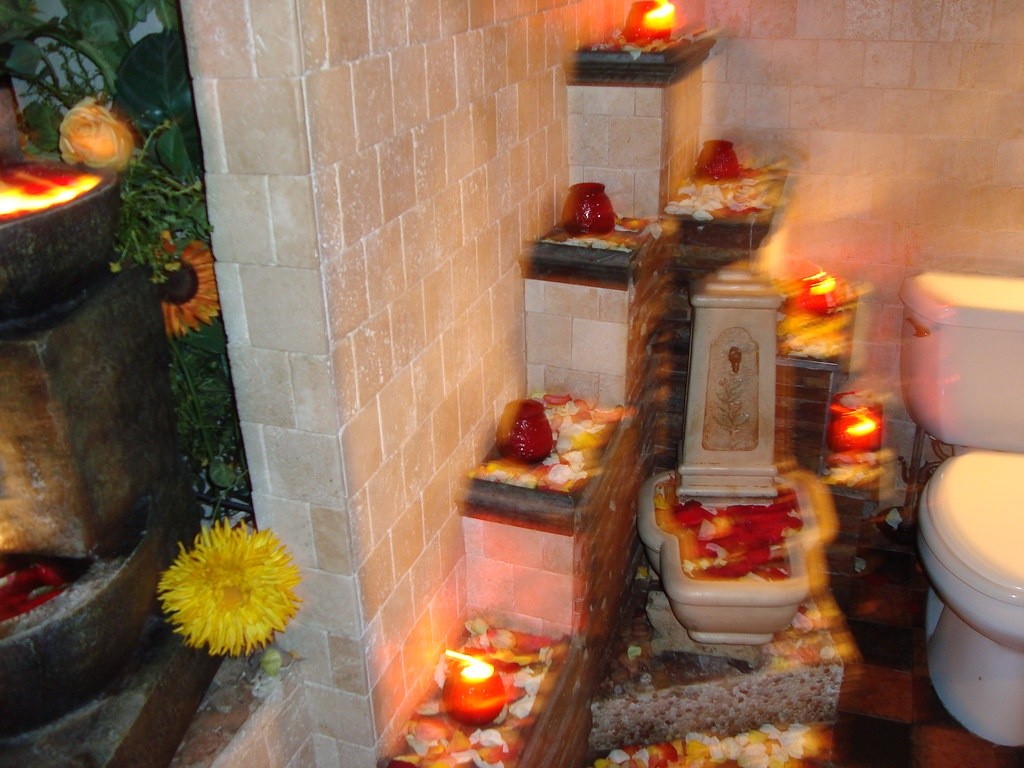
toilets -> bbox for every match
[897,267,1024,747]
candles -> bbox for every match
[443,650,506,727]
[561,183,615,238]
[626,0,675,44]
[802,276,836,316]
[497,398,552,466]
[696,140,738,180]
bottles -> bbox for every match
[496,400,552,464]
[562,183,615,237]
[695,139,739,180]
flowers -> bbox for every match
[59,96,136,174]
[157,516,302,659]
[152,236,221,340]
